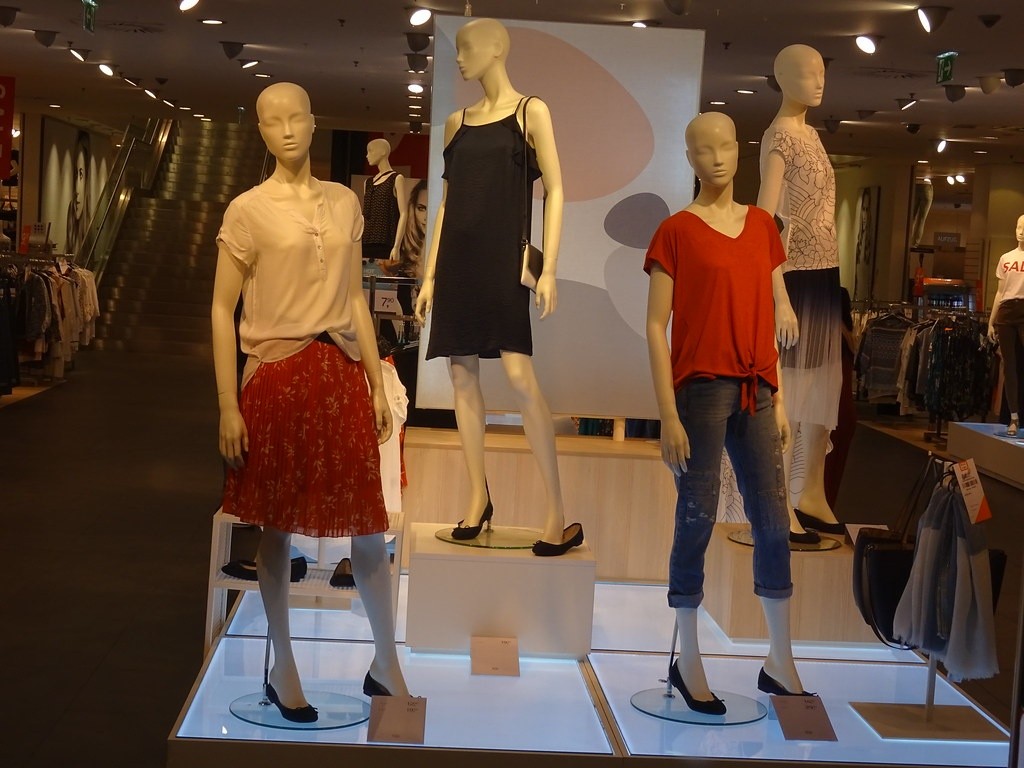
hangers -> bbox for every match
[919,309,987,334]
[849,296,906,323]
[0,253,81,276]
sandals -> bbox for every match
[1007,419,1020,435]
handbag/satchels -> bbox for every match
[520,243,543,294]
[0,199,17,222]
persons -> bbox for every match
[404,177,428,279]
[415,17,584,556]
[211,82,414,723]
[644,111,821,716]
[988,215,1024,435]
[757,45,848,545]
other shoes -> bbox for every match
[757,668,814,697]
[222,555,308,582]
[669,659,727,715]
[793,508,846,535]
[363,670,414,698]
[789,530,822,544]
[531,522,584,558]
[450,498,493,540]
[330,557,355,589]
[267,683,318,723]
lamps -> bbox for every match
[99,64,119,76]
[894,98,916,111]
[942,85,969,103]
[933,140,947,153]
[404,32,433,52]
[218,41,247,60]
[403,7,432,25]
[124,77,142,87]
[822,116,841,135]
[917,6,952,34]
[402,54,431,71]
[238,59,263,68]
[906,124,922,134]
[144,87,161,99]
[69,49,92,61]
[32,30,60,48]
[975,77,1004,95]
[1000,68,1024,89]
[409,122,422,133]
[856,36,885,54]
